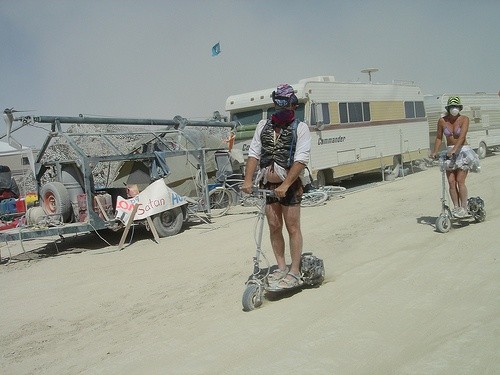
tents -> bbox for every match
[110,129,228,212]
[0,142,38,200]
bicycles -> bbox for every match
[203,172,346,218]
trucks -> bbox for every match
[224,75,430,190]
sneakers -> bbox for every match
[452,208,459,214]
[454,207,468,217]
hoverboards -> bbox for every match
[433,151,486,233]
[239,185,325,311]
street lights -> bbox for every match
[360,69,380,81]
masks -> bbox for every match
[449,107,460,116]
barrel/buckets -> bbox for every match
[77,190,113,222]
[0,193,37,213]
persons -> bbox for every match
[242,84,311,289]
[430,97,473,220]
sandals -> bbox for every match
[271,266,289,286]
[277,272,304,288]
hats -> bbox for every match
[272,84,298,107]
[445,97,463,111]
